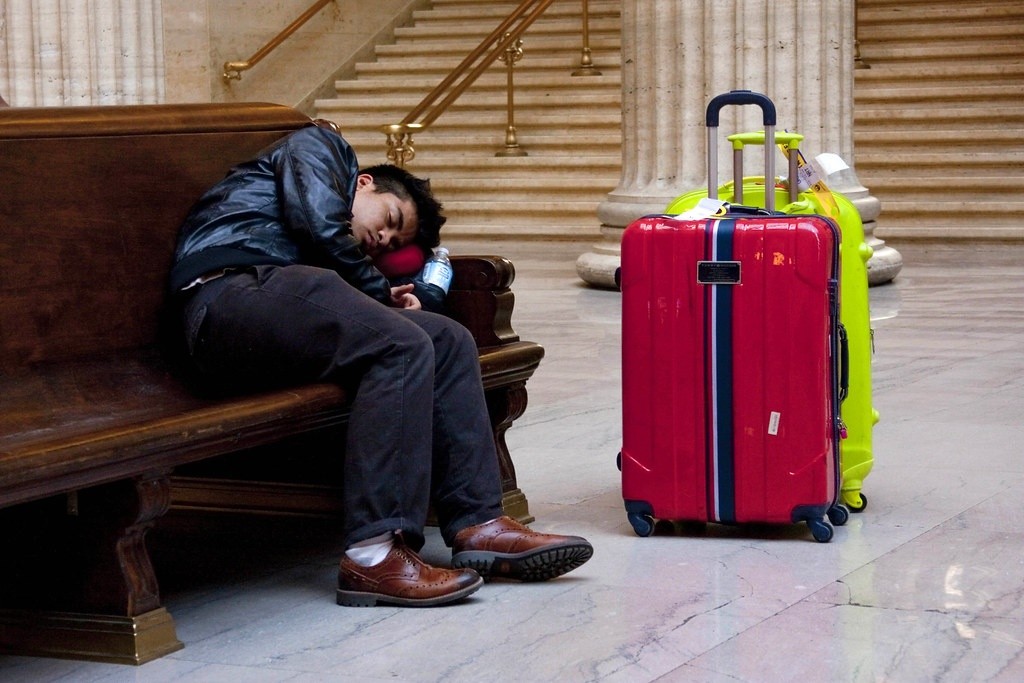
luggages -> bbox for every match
[619,89,879,542]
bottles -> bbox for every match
[422,248,453,295]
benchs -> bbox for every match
[1,99,545,668]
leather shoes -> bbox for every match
[336,535,485,608]
[452,515,593,582]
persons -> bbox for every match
[165,127,593,607]
